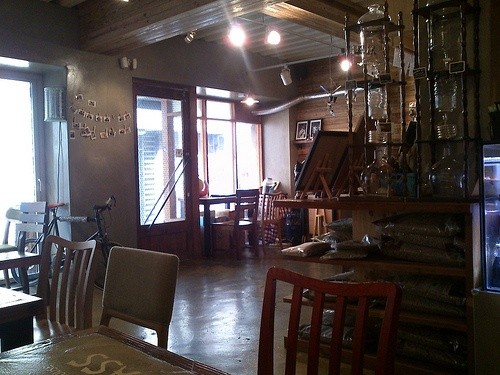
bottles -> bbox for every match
[262,178,273,195]
[357,2,469,198]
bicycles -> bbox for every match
[9,195,123,293]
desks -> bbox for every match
[0,288,42,352]
[0,326,229,375]
[200,196,240,260]
[0,242,39,270]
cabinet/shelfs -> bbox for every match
[273,196,480,375]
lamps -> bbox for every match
[280,64,292,87]
[184,31,196,44]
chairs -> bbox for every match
[0,189,401,375]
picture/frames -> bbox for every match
[309,119,323,140]
[295,120,309,141]
